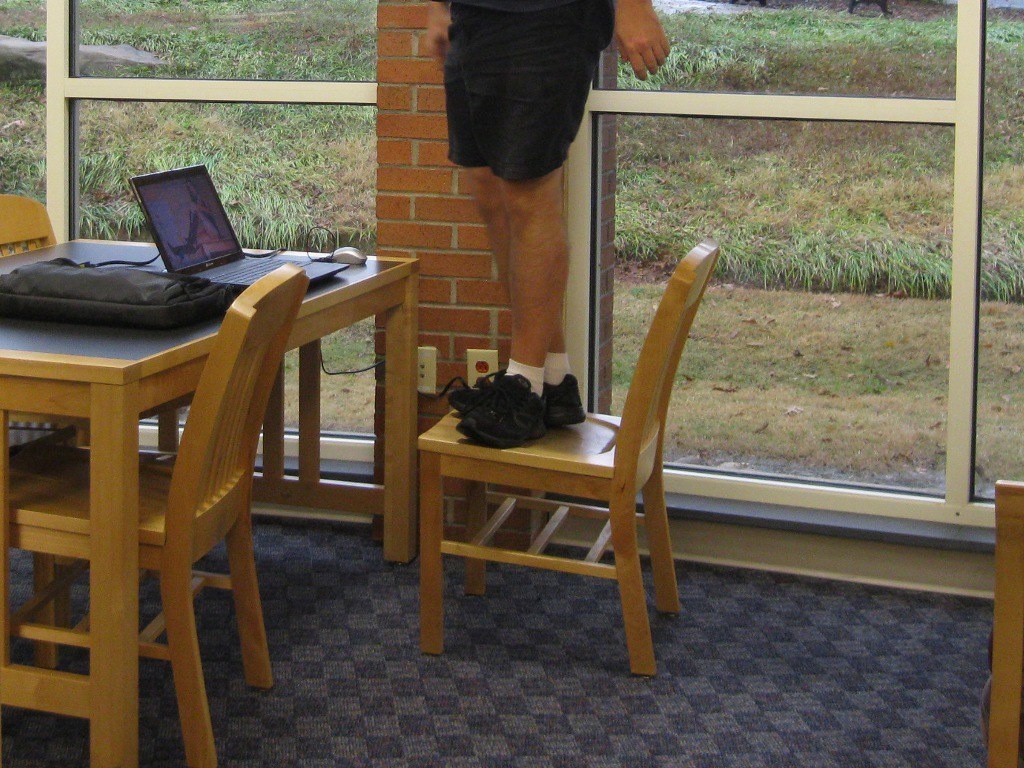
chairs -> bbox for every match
[1,195,87,451]
[2,262,309,768]
[417,237,716,677]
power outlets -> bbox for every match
[417,346,436,393]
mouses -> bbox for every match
[329,246,368,265]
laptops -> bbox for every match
[128,163,350,287]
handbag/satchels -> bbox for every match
[0,258,230,328]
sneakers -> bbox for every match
[448,370,586,425]
[456,374,548,447]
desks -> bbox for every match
[2,240,422,564]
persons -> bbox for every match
[167,179,221,258]
[421,0,671,449]
